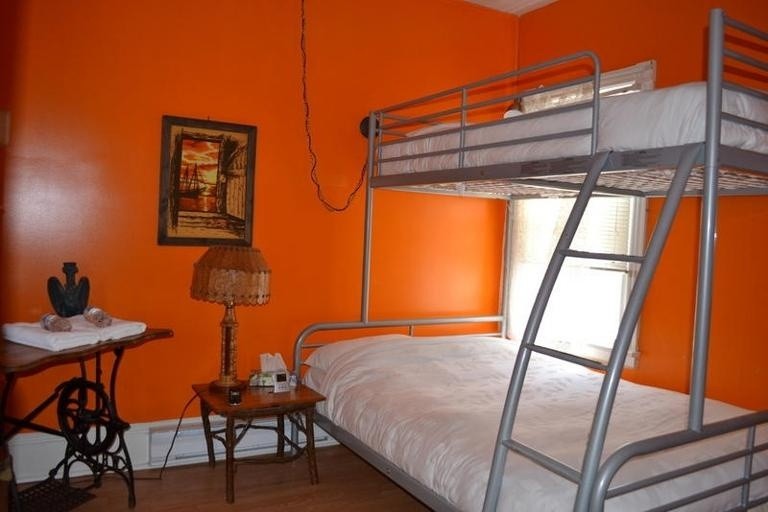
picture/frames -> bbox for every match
[159,113,256,247]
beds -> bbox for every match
[293,9,768,510]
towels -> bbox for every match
[67,313,146,341]
[1,321,100,353]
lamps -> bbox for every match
[187,244,272,395]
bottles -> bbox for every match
[83,306,112,328]
[40,312,72,332]
[230,390,240,405]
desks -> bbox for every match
[0,312,173,512]
[188,376,329,502]
